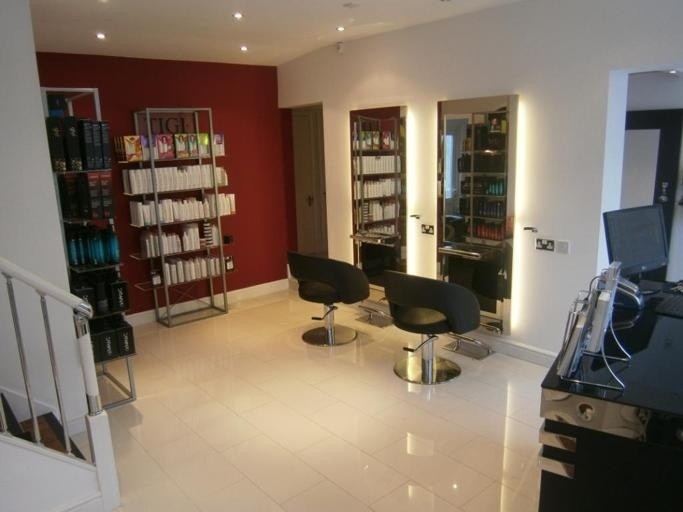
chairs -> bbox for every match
[286,249,371,348]
[385,267,482,385]
[359,237,400,287]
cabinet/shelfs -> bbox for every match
[352,114,400,231]
[38,87,137,413]
[455,107,505,243]
[115,107,229,328]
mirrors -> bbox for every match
[347,102,409,286]
[433,93,521,345]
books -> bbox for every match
[353,136,402,237]
[113,132,236,285]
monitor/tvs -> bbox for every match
[603,203,669,295]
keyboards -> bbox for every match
[654,294,683,318]
